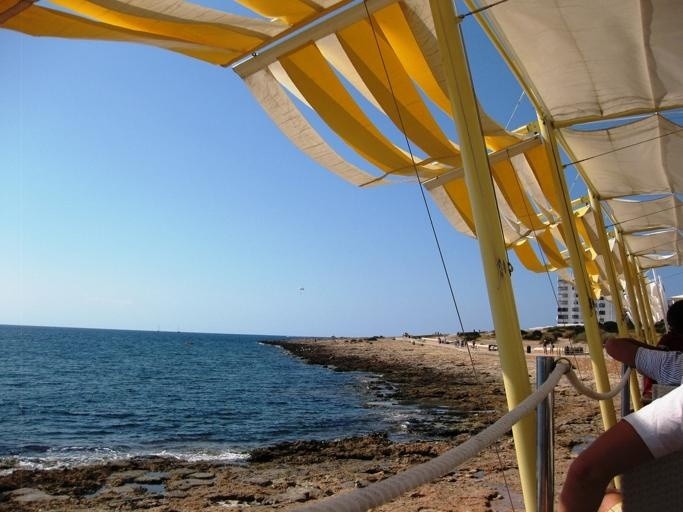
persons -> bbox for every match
[604,298,683,386]
[559,383,683,512]
